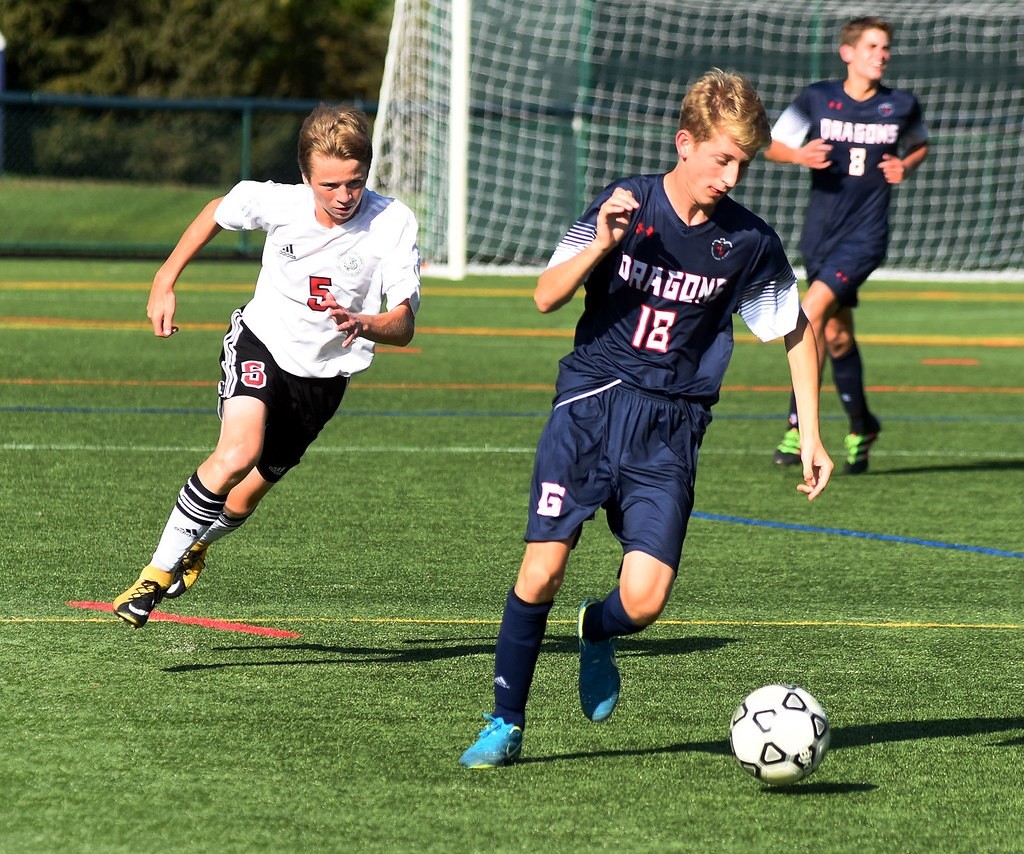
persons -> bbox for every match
[114,98,425,629]
[762,16,930,476]
[455,67,835,766]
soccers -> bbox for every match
[728,685,830,784]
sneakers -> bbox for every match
[456,712,523,769]
[772,427,802,467]
[577,597,621,723]
[164,540,208,599]
[112,563,175,629]
[843,414,880,475]
